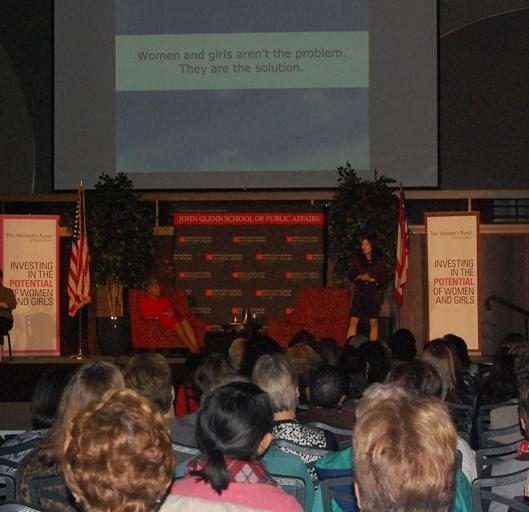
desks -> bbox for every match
[222,323,264,349]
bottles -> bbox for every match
[243,306,258,325]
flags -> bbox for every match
[67,187,92,317]
[393,190,412,305]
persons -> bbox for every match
[0,270,17,346]
[0,330,529,511]
[139,277,199,354]
[347,235,388,341]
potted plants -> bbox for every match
[75,172,161,355]
[327,160,407,336]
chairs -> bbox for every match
[1,332,13,362]
[0,396,529,512]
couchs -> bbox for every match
[127,289,206,352]
[269,287,352,349]
